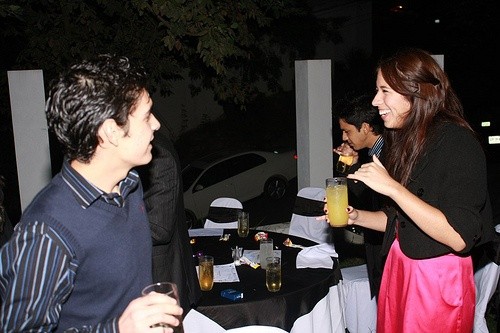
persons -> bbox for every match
[117,141,203,333]
[316,49,496,333]
[0,52,183,333]
[333,95,392,304]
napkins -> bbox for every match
[294,242,340,271]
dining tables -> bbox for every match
[185,229,343,333]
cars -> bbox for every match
[183,148,298,231]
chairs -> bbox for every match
[203,198,245,229]
[471,223,500,333]
[341,263,378,333]
[287,186,334,247]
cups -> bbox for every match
[199,257,213,290]
[337,147,354,174]
[142,282,184,333]
[266,257,281,292]
[260,238,273,269]
[238,211,249,237]
[326,178,349,227]
